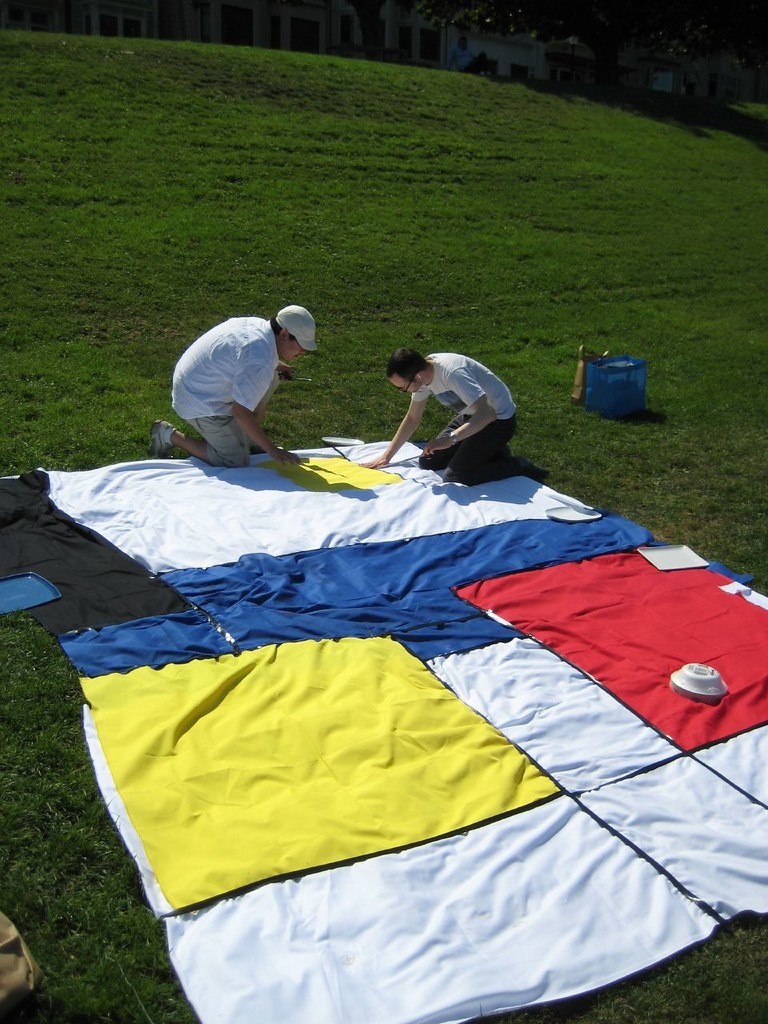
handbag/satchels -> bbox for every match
[571,345,609,405]
[586,356,647,418]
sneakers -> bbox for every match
[250,441,284,453]
[147,419,175,459]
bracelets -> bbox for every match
[447,431,457,446]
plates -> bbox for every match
[544,506,602,522]
[637,544,710,571]
[322,437,364,445]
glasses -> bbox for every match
[398,372,419,392]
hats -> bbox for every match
[276,304,318,350]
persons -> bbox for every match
[359,347,550,486]
[147,304,318,467]
